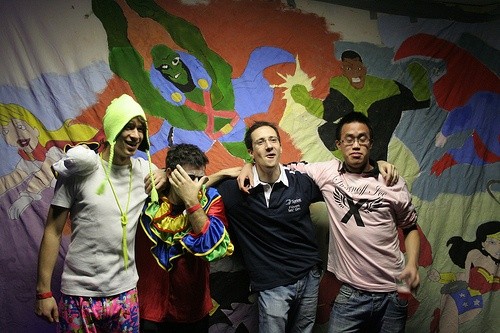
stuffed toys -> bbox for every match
[50,145,100,178]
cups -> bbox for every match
[397,276,409,306]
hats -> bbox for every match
[96,94,158,204]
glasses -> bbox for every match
[251,137,280,146]
[162,167,210,185]
[339,135,373,146]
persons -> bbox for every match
[135,144,236,333]
[35,94,249,332]
[143,120,400,332]
[236,111,422,333]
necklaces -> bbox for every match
[99,152,133,273]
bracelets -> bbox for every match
[186,203,201,215]
[35,291,53,299]
